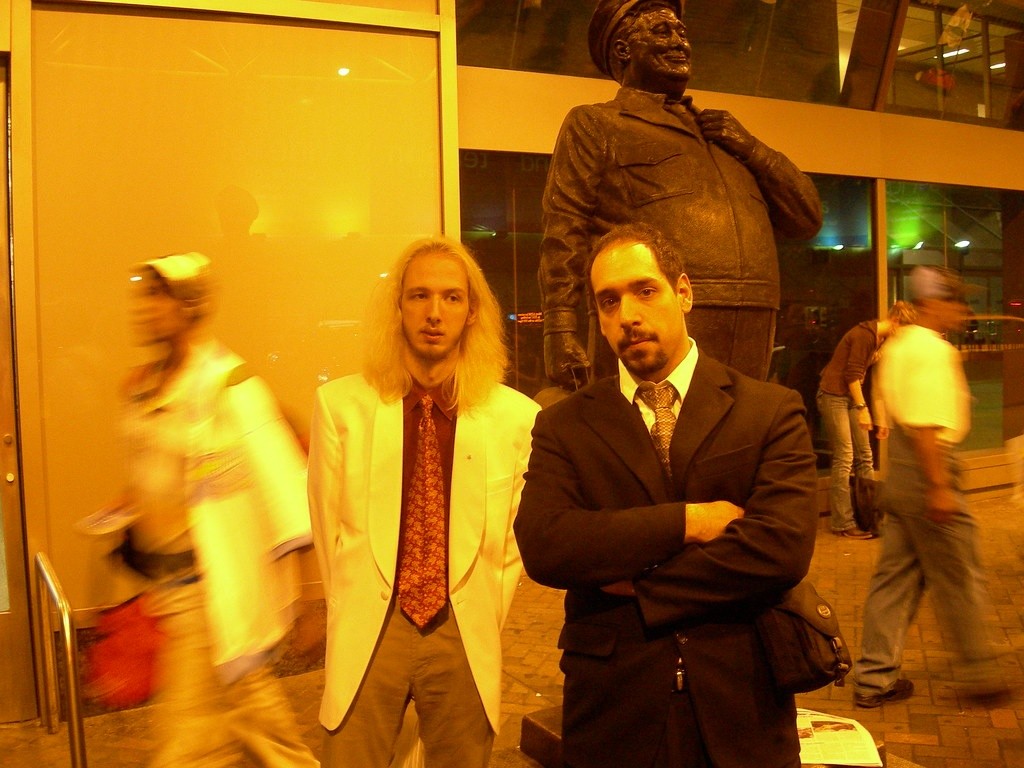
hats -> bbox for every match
[130,250,219,300]
[910,263,984,298]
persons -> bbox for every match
[307,237,544,768]
[960,301,979,345]
[536,1,824,387]
[511,223,820,768]
[771,302,821,446]
[88,250,325,768]
[816,264,1020,709]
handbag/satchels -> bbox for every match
[849,475,888,537]
[755,580,853,695]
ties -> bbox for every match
[398,394,447,628]
[636,381,678,483]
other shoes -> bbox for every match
[856,678,914,708]
[833,528,872,540]
